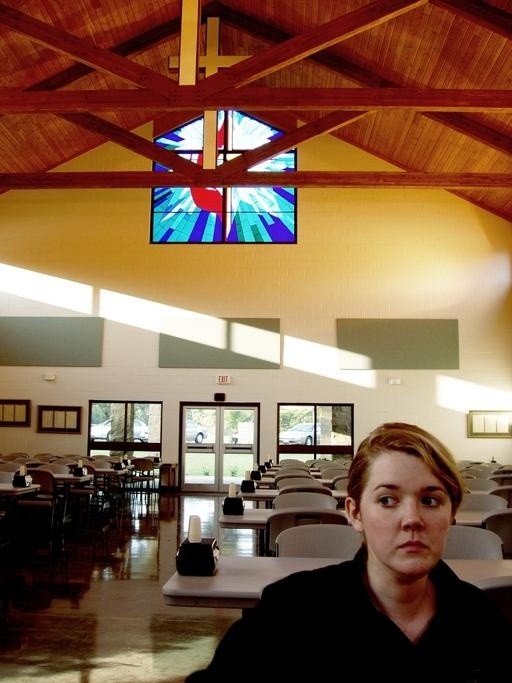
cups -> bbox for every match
[78,460,82,467]
[229,482,236,497]
[119,456,123,462]
[188,515,203,543]
[20,464,27,475]
[245,469,250,480]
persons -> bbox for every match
[179,422,511,682]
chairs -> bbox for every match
[0,454,161,611]
[264,458,512,590]
